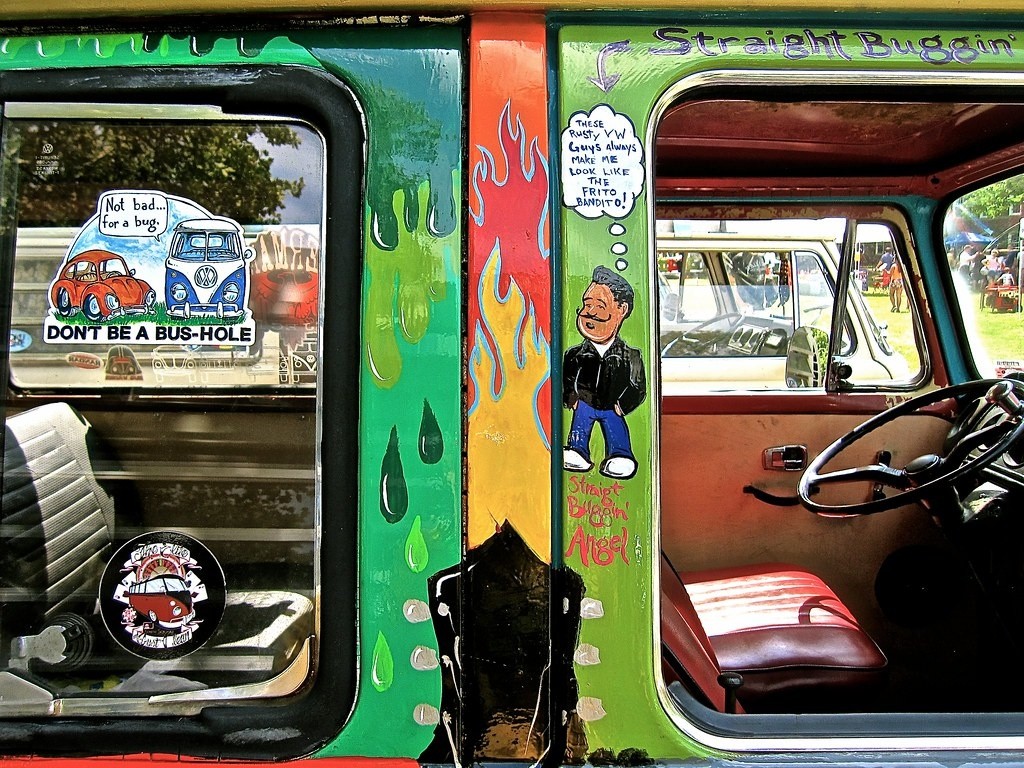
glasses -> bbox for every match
[992,251,997,254]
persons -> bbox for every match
[876,247,916,312]
[947,239,1024,293]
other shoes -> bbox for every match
[896,310,900,313]
[891,306,897,312]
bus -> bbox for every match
[14,219,907,392]
[2,0,1024,768]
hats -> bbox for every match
[990,248,998,254]
[964,245,973,250]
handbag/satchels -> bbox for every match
[980,265,989,276]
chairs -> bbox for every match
[656,547,889,714]
[872,269,890,296]
[0,402,315,683]
